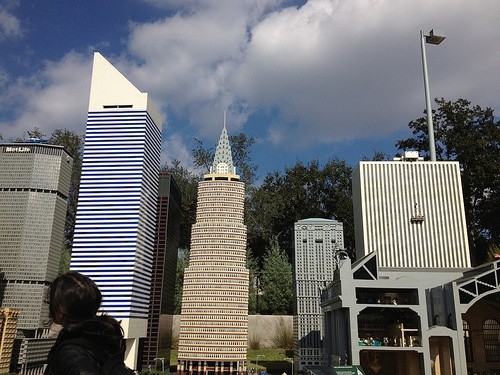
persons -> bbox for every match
[41,271,134,375]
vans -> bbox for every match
[331,365,365,375]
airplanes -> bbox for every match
[25,130,49,144]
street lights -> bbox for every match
[284,357,294,375]
[153,358,164,372]
[255,355,265,375]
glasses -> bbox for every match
[42,295,54,307]
